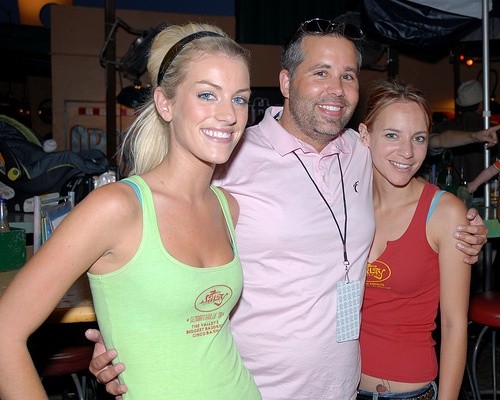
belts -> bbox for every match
[356,387,434,400]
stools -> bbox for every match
[461,288,500,400]
[28,327,105,400]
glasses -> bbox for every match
[283,18,367,54]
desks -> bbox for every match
[0,245,97,325]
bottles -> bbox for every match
[0,198,10,233]
[419,160,499,209]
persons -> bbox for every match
[83,16,485,400]
[0,16,273,400]
[350,77,471,399]
[427,78,500,211]
[421,121,499,159]
